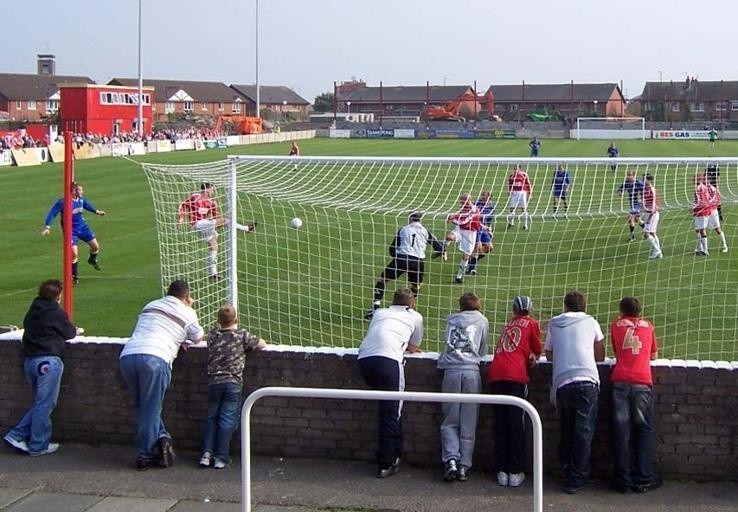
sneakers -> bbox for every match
[508,223,515,227]
[245,223,257,233]
[458,465,473,481]
[564,213,567,218]
[209,273,224,279]
[523,226,528,230]
[633,480,662,493]
[612,481,633,494]
[650,250,662,259]
[564,481,585,494]
[88,258,101,271]
[571,468,601,487]
[72,275,79,287]
[468,270,476,275]
[376,467,394,478]
[135,454,161,471]
[29,443,60,457]
[720,248,728,252]
[432,253,442,258]
[159,437,176,467]
[497,472,508,485]
[443,458,457,481]
[364,308,375,319]
[4,436,29,455]
[200,452,211,465]
[552,215,558,219]
[695,249,700,255]
[509,472,525,486]
[700,250,709,256]
[393,458,399,474]
[626,238,636,243]
[213,457,232,469]
[456,272,464,282]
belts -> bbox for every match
[563,384,593,388]
[631,384,649,388]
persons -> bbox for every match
[366,214,444,320]
[709,128,718,148]
[705,155,724,220]
[550,166,570,218]
[0,123,227,152]
[119,281,204,472]
[639,174,663,258]
[468,193,496,275]
[490,296,542,487]
[618,172,647,241]
[696,174,729,254]
[290,142,300,171]
[357,289,424,478]
[607,141,618,171]
[42,182,106,284]
[5,280,84,456]
[445,194,480,282]
[610,297,663,493]
[528,137,541,157]
[507,164,532,230]
[198,305,266,468]
[545,292,606,492]
[437,294,489,482]
[689,175,709,255]
[178,183,257,281]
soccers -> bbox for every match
[291,218,302,229]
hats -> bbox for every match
[513,296,533,316]
